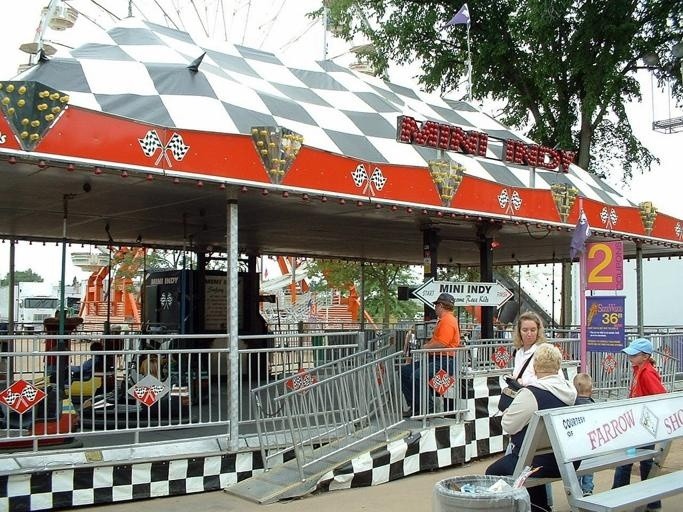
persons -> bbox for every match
[41,308,83,376]
[468,312,504,359]
[401,324,419,363]
[64,342,105,386]
[484,342,578,511]
[498,311,554,511]
[396,292,459,417]
[611,338,669,512]
[137,358,161,383]
[567,372,596,499]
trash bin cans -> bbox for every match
[312,328,408,368]
[436,474,519,512]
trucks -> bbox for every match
[0,281,63,334]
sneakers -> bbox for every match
[401,408,418,418]
[582,489,593,498]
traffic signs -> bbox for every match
[411,276,516,312]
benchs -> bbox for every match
[496,392,676,512]
[544,390,682,512]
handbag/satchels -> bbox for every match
[497,374,524,412]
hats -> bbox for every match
[431,292,455,309]
[621,337,654,357]
